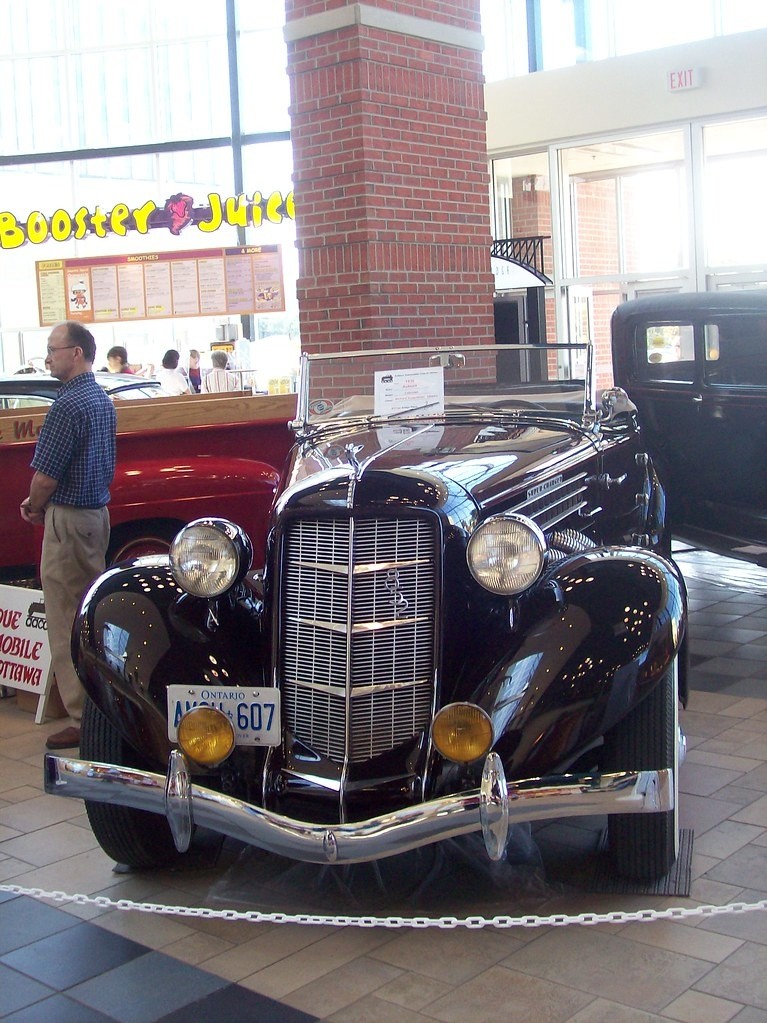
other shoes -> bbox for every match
[46,727,79,749]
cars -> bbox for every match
[46,340,689,880]
[0,371,303,624]
[593,288,767,569]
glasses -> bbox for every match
[48,346,84,353]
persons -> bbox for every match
[200,350,241,394]
[20,323,118,750]
[107,346,136,375]
[178,350,208,393]
[156,350,192,397]
